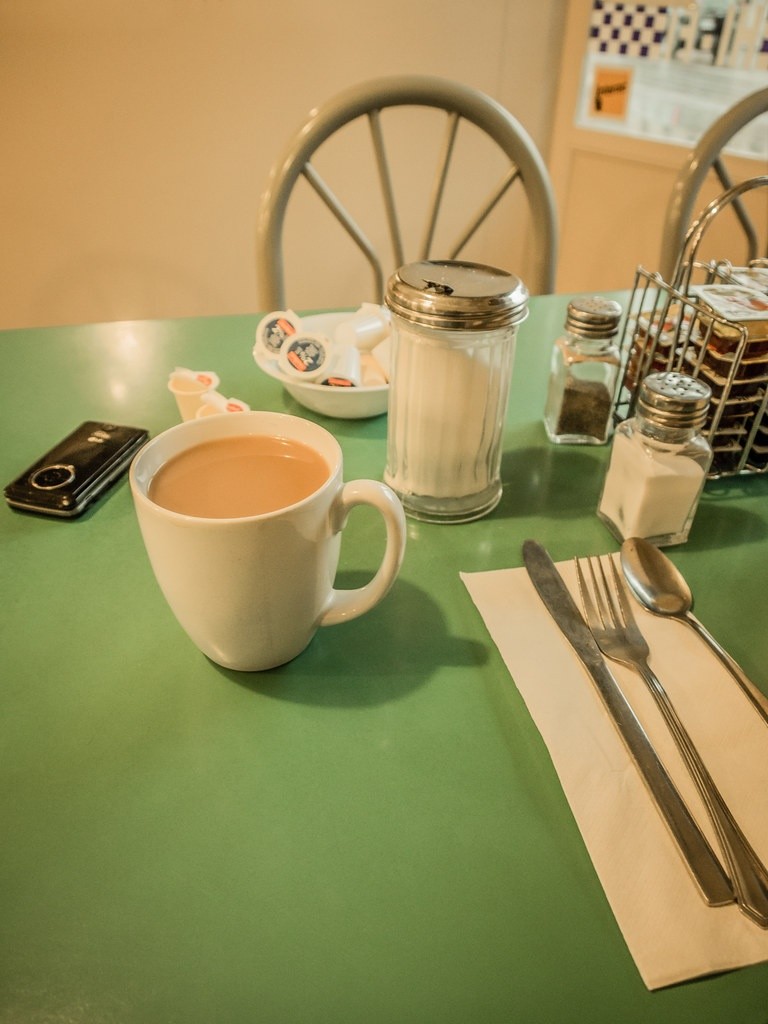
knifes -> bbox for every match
[520,540,735,908]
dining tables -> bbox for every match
[0,292,768,1023]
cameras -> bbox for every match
[3,421,150,518]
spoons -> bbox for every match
[619,538,768,727]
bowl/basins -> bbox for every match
[253,312,389,419]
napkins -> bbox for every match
[459,550,768,990]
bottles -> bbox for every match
[597,373,713,550]
[542,295,621,445]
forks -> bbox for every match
[574,552,768,930]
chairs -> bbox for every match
[659,86,767,287]
[256,68,559,322]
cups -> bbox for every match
[128,412,408,673]
[385,260,530,524]
